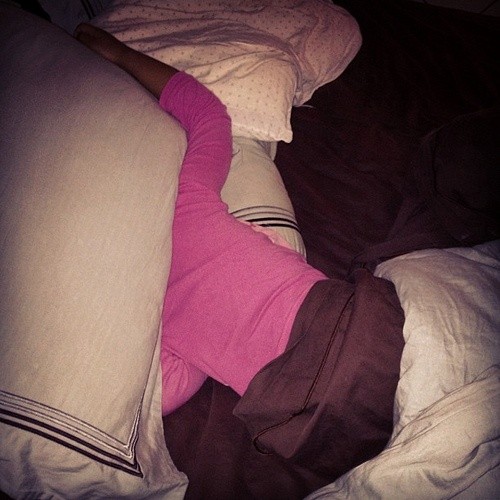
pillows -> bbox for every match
[0,1,189,480]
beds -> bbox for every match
[0,1,500,500]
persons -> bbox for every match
[73,23,406,493]
